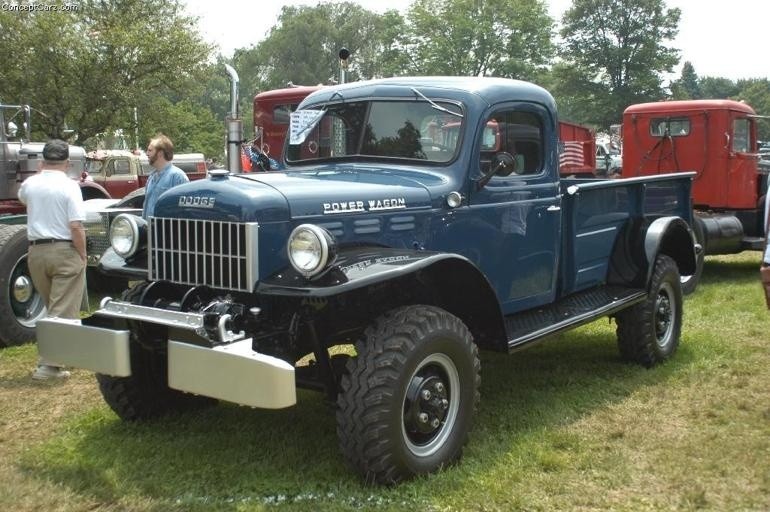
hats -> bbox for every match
[42,139,68,160]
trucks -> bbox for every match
[0,46,770,346]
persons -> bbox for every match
[141,134,190,222]
[17,139,87,381]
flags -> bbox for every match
[559,140,584,167]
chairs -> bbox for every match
[513,138,541,171]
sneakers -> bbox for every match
[32,365,71,380]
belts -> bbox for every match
[28,238,71,245]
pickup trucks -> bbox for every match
[33,61,708,488]
